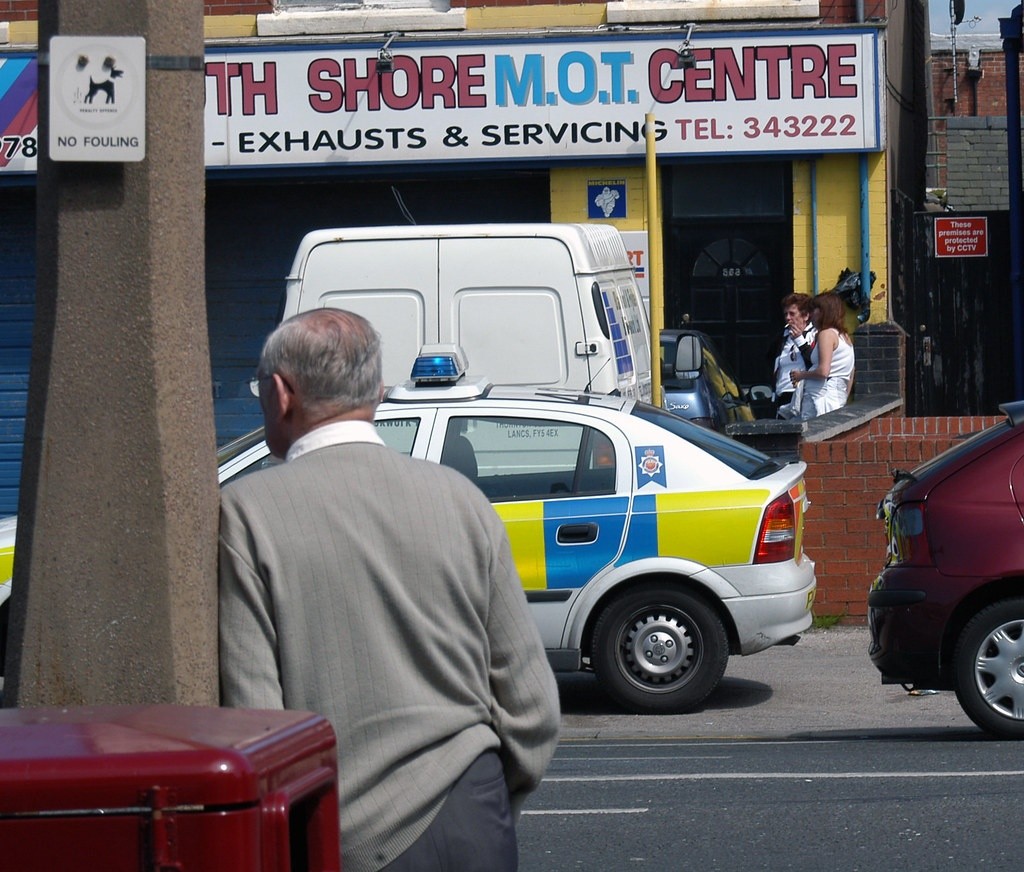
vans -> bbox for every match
[284,224,656,406]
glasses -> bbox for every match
[247,373,296,397]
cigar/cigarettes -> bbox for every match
[784,323,790,328]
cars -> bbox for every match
[2,346,817,711]
[659,328,773,434]
[866,401,1024,738]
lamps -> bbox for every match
[677,22,697,69]
[375,31,405,73]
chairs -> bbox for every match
[444,421,479,483]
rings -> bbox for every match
[788,330,793,334]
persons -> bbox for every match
[772,291,820,421]
[789,288,857,423]
[215,305,565,870]
